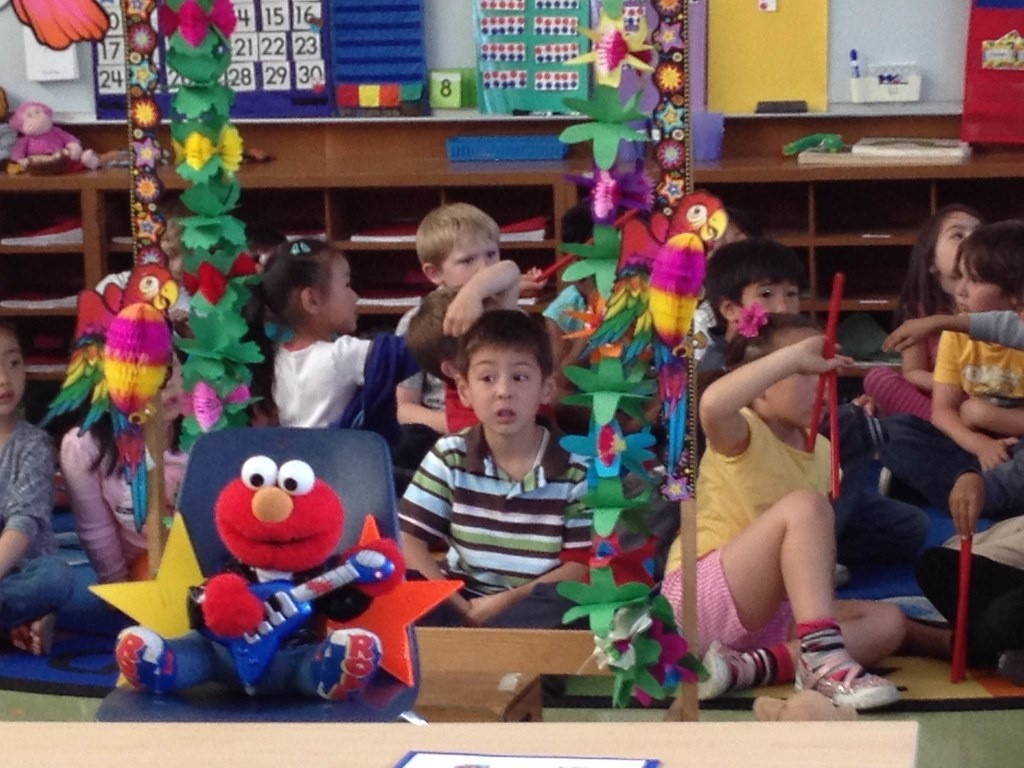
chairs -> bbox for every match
[95,429,427,725]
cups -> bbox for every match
[690,113,723,162]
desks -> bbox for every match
[0,719,919,768]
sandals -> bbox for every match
[10,613,57,655]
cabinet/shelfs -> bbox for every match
[0,113,1023,448]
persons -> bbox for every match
[0,322,188,655]
[399,307,591,628]
[689,207,1024,683]
[660,313,909,711]
[261,204,657,470]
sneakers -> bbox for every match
[793,649,898,709]
[697,638,754,700]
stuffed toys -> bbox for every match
[116,455,404,700]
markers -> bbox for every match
[850,49,860,78]
[513,109,561,116]
